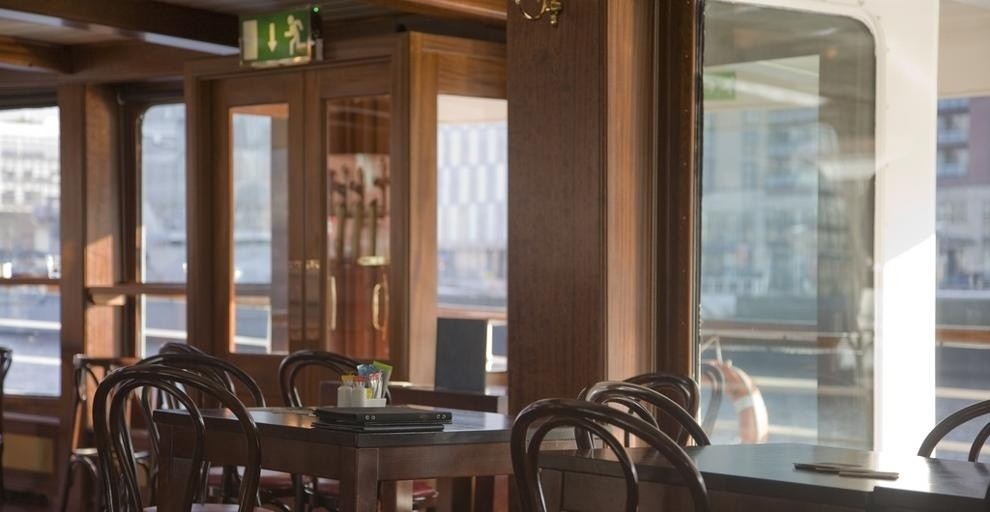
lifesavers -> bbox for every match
[700,359,768,444]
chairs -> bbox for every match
[60,338,988,512]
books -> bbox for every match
[311,422,445,432]
